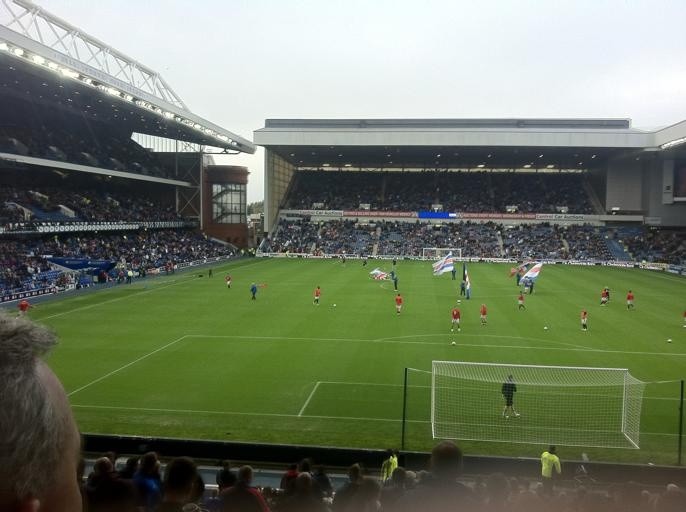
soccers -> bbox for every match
[544,327,548,330]
[333,304,336,307]
[451,342,456,346]
[667,339,672,343]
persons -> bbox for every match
[451,267,456,280]
[0,311,87,511]
[479,304,487,327]
[0,92,258,304]
[392,275,400,291]
[517,290,525,312]
[626,289,634,311]
[75,439,686,511]
[449,306,461,333]
[528,279,535,294]
[460,280,467,296]
[599,288,607,307]
[580,309,588,332]
[522,278,530,294]
[393,292,402,316]
[15,298,37,319]
[499,375,520,421]
[388,270,395,279]
[258,168,685,268]
[606,288,610,301]
[312,285,321,306]
[516,270,521,286]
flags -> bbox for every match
[367,268,388,280]
[509,262,529,277]
[430,250,454,277]
[463,263,471,301]
[518,261,542,286]
